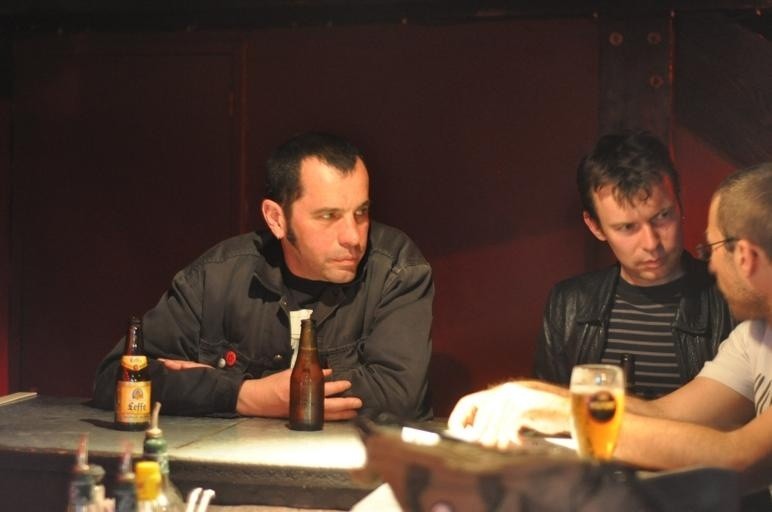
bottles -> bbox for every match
[69,403,187,511]
[290,318,324,431]
[114,315,154,433]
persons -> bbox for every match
[91,129,435,422]
[540,128,737,401]
[446,160,772,473]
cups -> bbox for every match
[568,363,625,470]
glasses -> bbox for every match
[696,234,729,260]
